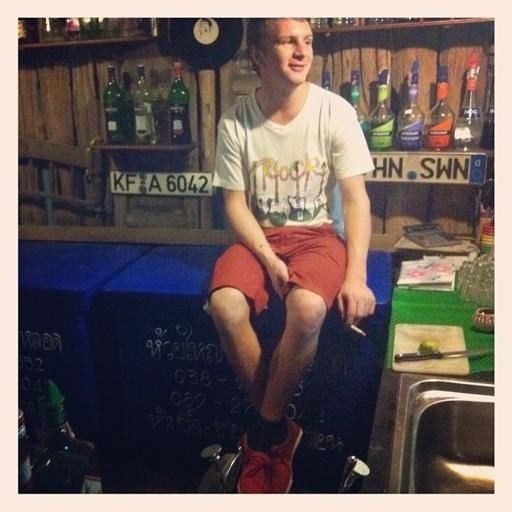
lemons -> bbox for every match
[419,339,439,356]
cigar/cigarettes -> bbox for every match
[349,325,367,337]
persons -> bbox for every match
[209,17,376,493]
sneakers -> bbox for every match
[238,416,303,493]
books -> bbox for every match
[396,259,456,292]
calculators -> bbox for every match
[403,223,462,248]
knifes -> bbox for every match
[392,349,490,363]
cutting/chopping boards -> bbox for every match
[392,324,470,377]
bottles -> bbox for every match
[321,59,482,153]
[19,19,109,44]
[168,62,191,145]
[132,64,159,144]
[102,67,123,143]
